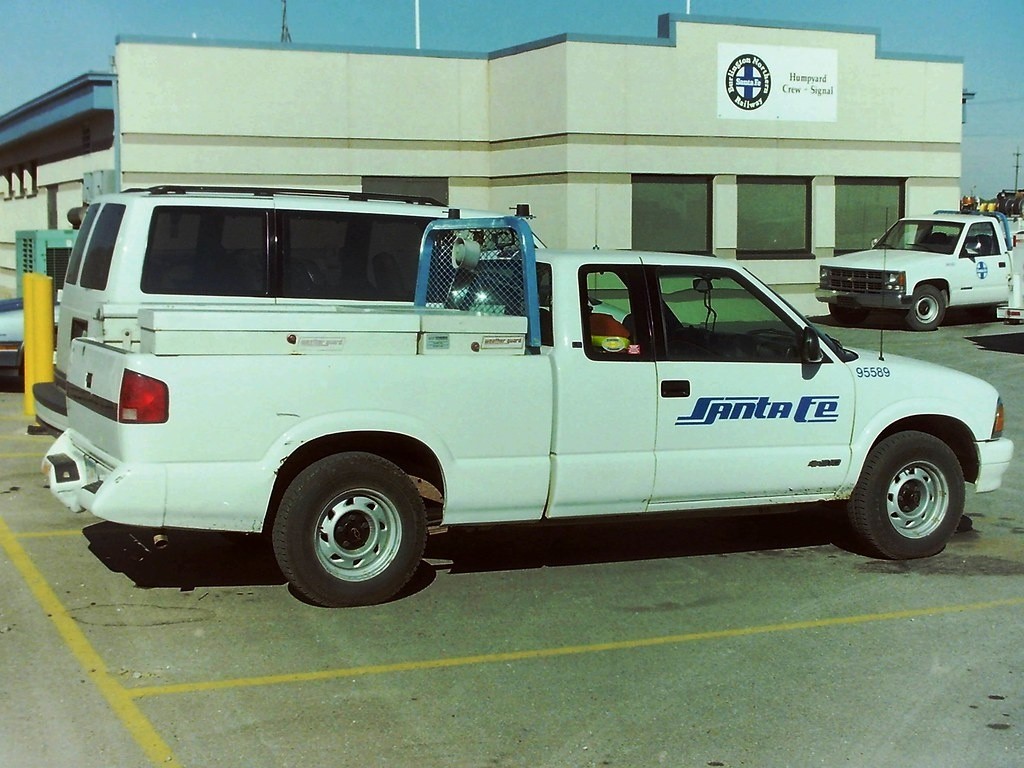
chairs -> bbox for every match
[926,232,996,255]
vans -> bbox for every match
[32,186,549,434]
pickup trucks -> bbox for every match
[43,216,1013,608]
[816,211,1023,330]
